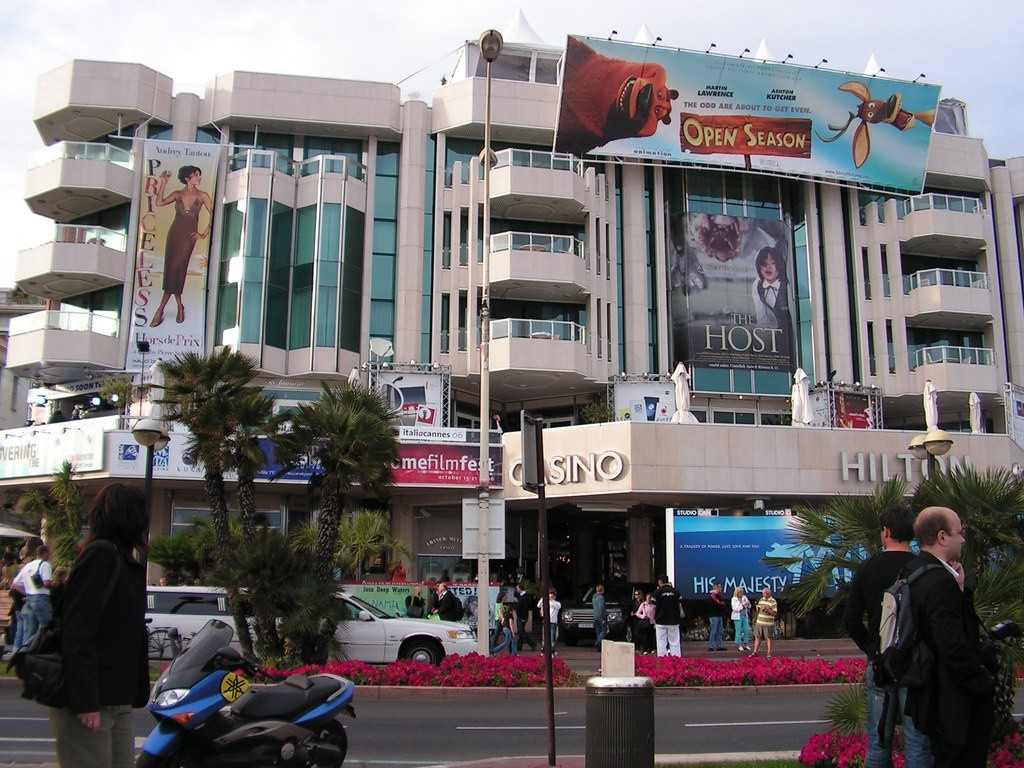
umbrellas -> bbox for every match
[969,392,981,433]
[671,362,699,423]
[0,523,38,537]
[924,379,938,430]
[791,368,814,426]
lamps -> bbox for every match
[913,73,926,82]
[706,44,716,53]
[817,380,878,389]
[360,359,439,372]
[609,30,618,40]
[783,54,793,63]
[652,37,662,46]
[873,67,886,77]
[620,371,670,379]
[815,59,828,68]
[739,48,750,57]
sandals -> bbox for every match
[767,651,771,657]
[749,652,758,657]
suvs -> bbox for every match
[561,582,656,642]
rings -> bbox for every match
[89,722,94,723]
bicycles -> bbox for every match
[144,618,181,660]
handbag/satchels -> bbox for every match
[407,606,422,616]
[428,613,440,620]
[31,560,46,589]
[639,621,649,628]
[680,603,685,618]
[540,610,544,617]
[16,652,76,707]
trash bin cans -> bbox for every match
[586,676,655,768]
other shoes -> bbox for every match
[738,647,743,651]
[651,651,655,654]
[743,645,751,650]
[708,648,713,652]
[593,644,601,651]
[644,652,647,655]
[717,647,728,651]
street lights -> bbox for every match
[907,429,954,482]
[130,417,171,572]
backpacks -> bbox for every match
[873,563,943,690]
[454,598,464,620]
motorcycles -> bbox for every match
[134,618,356,768]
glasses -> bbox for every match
[948,529,965,537]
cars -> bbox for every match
[146,586,479,667]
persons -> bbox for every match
[492,412,507,431]
[592,585,609,651]
[0,537,69,653]
[626,575,685,656]
[389,560,406,581]
[752,247,792,328]
[490,584,560,656]
[48,409,80,424]
[159,578,201,586]
[33,484,150,768]
[459,596,478,622]
[843,506,933,768]
[150,166,213,326]
[708,583,778,657]
[431,581,458,621]
[900,506,1000,768]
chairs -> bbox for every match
[927,353,938,363]
[963,356,973,363]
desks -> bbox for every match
[938,357,958,363]
[519,244,550,252]
[529,332,551,339]
